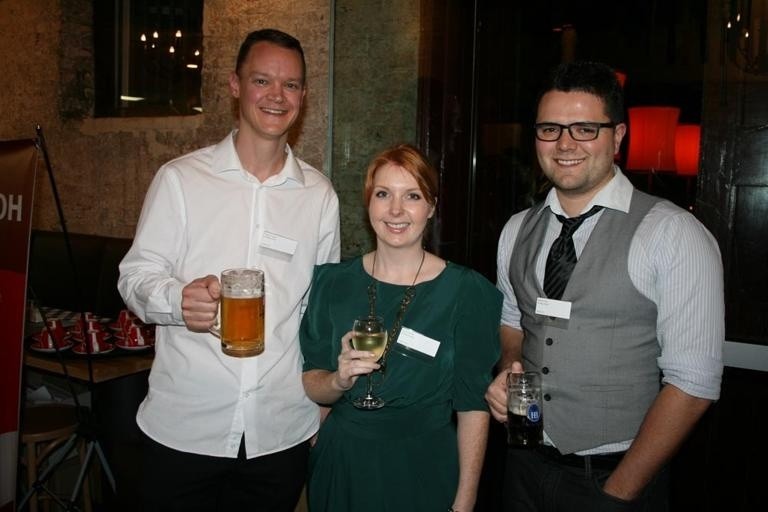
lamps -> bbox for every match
[625,105,683,194]
[671,121,704,201]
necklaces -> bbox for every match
[366,248,425,376]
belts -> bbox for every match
[544,452,631,471]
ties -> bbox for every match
[544,206,604,299]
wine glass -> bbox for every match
[353,316,388,409]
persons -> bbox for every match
[118,30,340,512]
[298,144,505,511]
[485,64,726,510]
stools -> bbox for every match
[19,404,94,511]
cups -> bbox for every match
[32,309,150,355]
[506,373,543,448]
[206,268,266,356]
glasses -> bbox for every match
[533,122,613,142]
